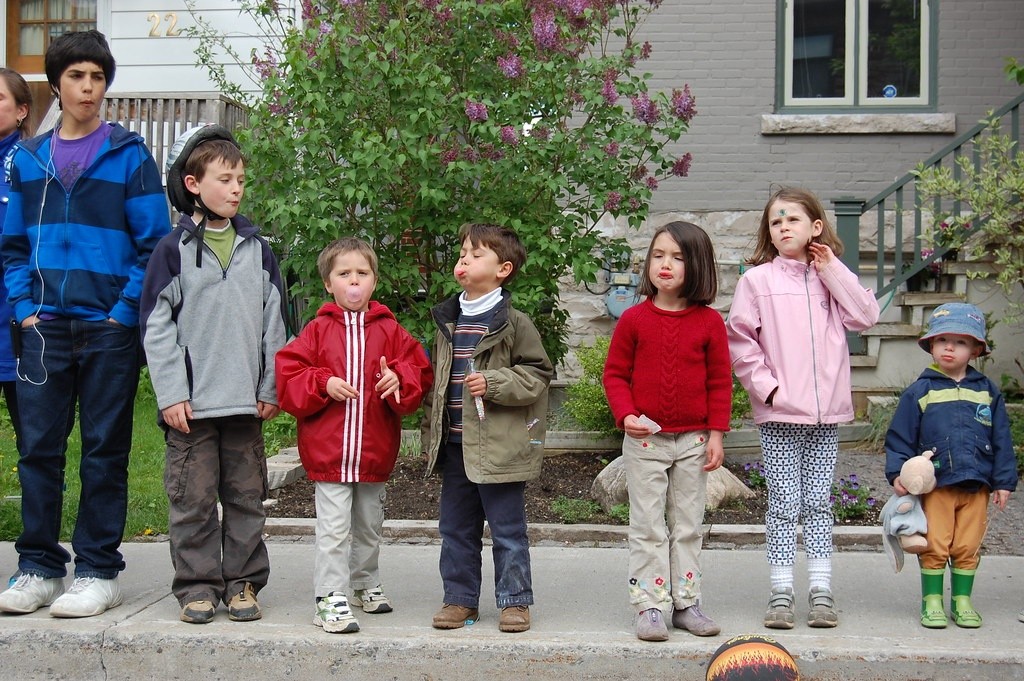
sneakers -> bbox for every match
[0,573,68,612]
[807,586,836,627]
[227,581,262,621]
[313,591,360,633]
[350,582,393,613]
[49,575,123,618]
[432,602,480,628]
[765,586,794,628]
[498,602,530,631]
[180,599,216,623]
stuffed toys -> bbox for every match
[878,451,937,573]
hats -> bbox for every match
[917,303,990,358]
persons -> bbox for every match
[884,303,1018,629]
[274,238,434,634]
[0,29,173,619]
[421,224,553,633]
[725,186,880,629]
[139,125,291,625]
[602,221,732,641]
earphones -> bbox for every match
[55,86,59,95]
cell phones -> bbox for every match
[12,321,21,359]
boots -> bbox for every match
[919,568,947,628]
[949,566,982,627]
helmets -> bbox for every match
[165,122,236,215]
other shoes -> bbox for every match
[672,600,721,636]
[636,607,670,641]
